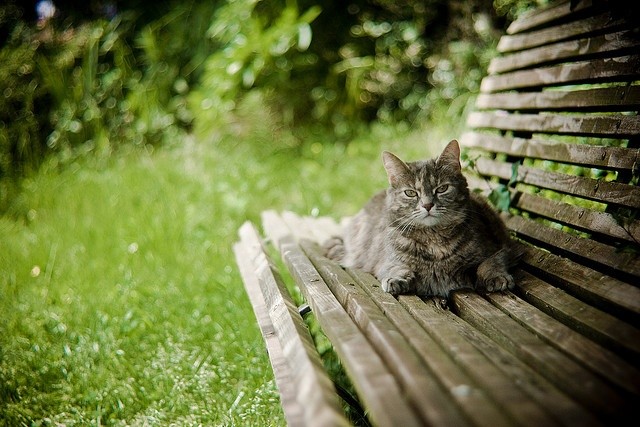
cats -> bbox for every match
[325,139,515,299]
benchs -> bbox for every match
[233,0,639,426]
